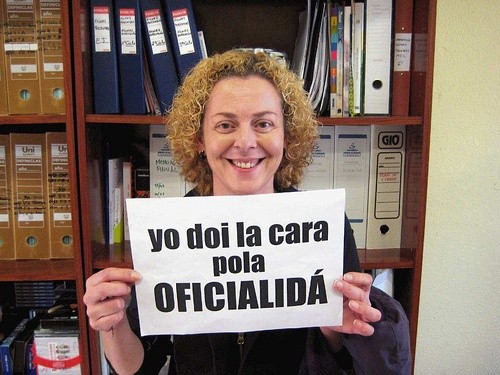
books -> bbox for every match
[105,158,133,262]
[330,0,414,118]
[0,282,81,375]
[198,30,208,62]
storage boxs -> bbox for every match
[34,325,81,375]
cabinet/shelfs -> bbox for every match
[71,0,438,374]
[0,0,90,375]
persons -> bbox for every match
[83,49,412,375]
